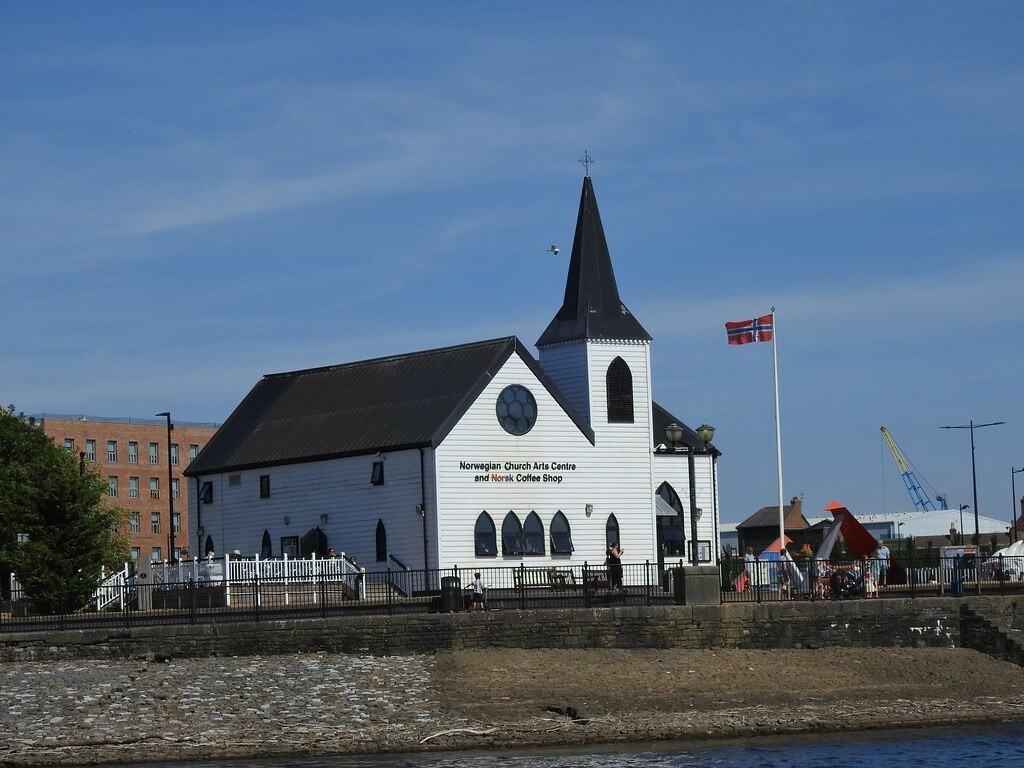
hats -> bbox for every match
[866,571,871,577]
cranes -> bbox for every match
[881,426,949,514]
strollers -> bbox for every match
[828,568,866,600]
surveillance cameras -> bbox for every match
[660,446,667,452]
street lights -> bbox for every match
[940,421,1005,543]
[664,423,716,567]
[898,522,904,549]
[960,504,970,546]
[730,547,738,574]
[167,533,177,566]
[154,412,176,566]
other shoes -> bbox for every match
[883,584,887,587]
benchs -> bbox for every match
[548,570,577,592]
[582,569,611,591]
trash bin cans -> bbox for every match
[442,575,464,611]
[950,581,963,598]
[674,565,723,607]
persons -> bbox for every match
[990,563,998,581]
[744,547,756,592]
[869,551,880,597]
[776,548,794,604]
[328,548,335,556]
[863,571,877,598]
[606,541,624,593]
[854,560,862,572]
[861,554,871,576]
[806,558,837,599]
[350,556,357,565]
[875,540,891,587]
[464,573,486,613]
[830,575,850,595]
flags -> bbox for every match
[725,314,773,344]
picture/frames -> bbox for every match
[688,540,712,563]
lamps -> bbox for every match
[586,504,593,514]
[320,514,328,524]
[197,526,205,536]
[415,504,424,517]
[283,516,290,525]
[697,507,702,521]
